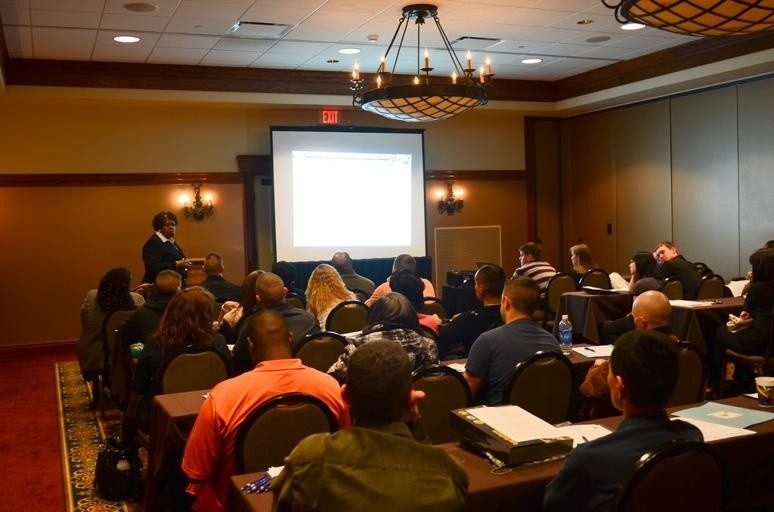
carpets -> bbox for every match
[54,357,150,512]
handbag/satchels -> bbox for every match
[93,435,141,504]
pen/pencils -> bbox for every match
[240,472,271,494]
[583,436,588,442]
[585,348,596,352]
[757,383,772,401]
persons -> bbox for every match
[77,211,772,512]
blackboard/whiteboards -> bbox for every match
[434,224,503,300]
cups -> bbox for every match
[755,376,774,408]
[129,343,143,363]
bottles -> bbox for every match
[558,316,573,355]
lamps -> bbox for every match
[177,179,215,222]
[600,0,774,42]
[435,180,465,216]
[350,3,495,123]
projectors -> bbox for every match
[447,271,475,287]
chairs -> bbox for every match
[91,268,773,511]
